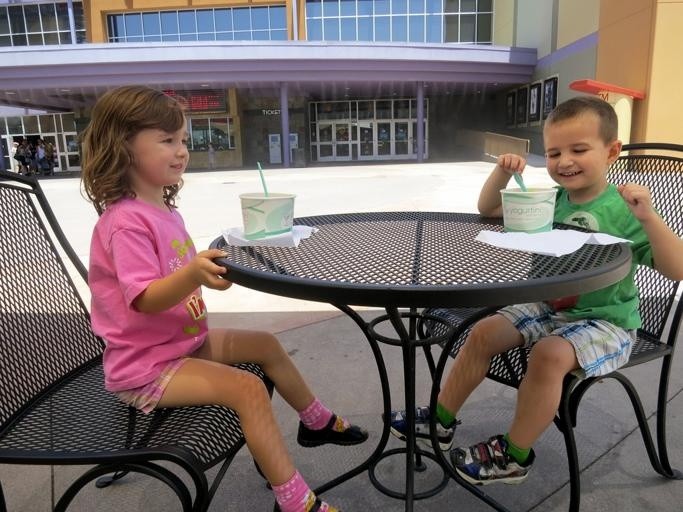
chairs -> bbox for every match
[408,139,683,511]
[1,142,279,512]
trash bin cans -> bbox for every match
[292,147,306,167]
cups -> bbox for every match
[498,188,559,232]
[239,192,296,239]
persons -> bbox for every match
[380,94,683,486]
[75,83,368,512]
[12,138,55,178]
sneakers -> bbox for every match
[381,406,458,452]
[450,433,536,486]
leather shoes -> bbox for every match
[296,415,369,447]
[272,496,322,511]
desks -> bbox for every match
[209,206,631,512]
